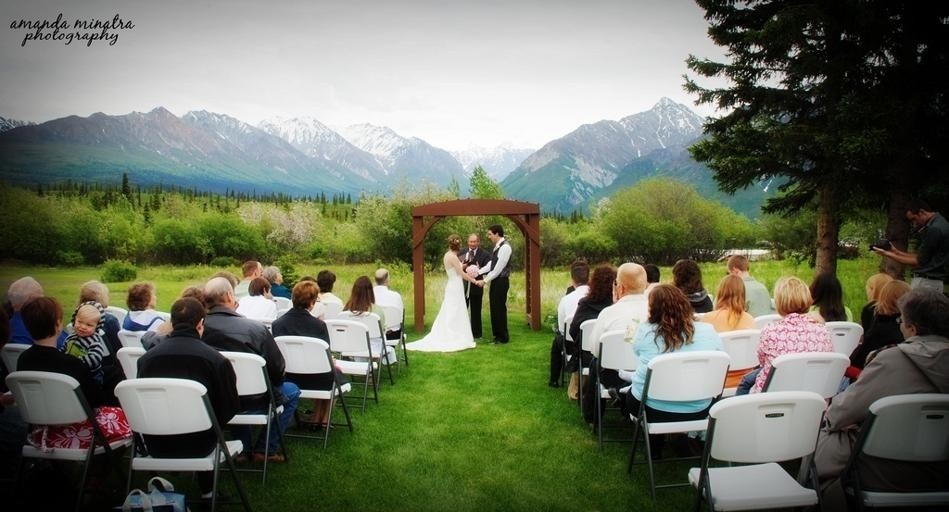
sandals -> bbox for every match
[321,421,336,431]
[311,423,319,432]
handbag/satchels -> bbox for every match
[567,372,587,400]
[115,477,186,512]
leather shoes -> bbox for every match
[549,382,557,387]
[235,453,250,462]
[253,454,286,463]
[200,491,231,502]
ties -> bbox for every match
[468,250,474,261]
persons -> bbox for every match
[458,234,492,339]
[478,224,513,345]
[871,198,949,293]
[552,254,949,508]
[401,234,476,352]
[0,261,404,512]
